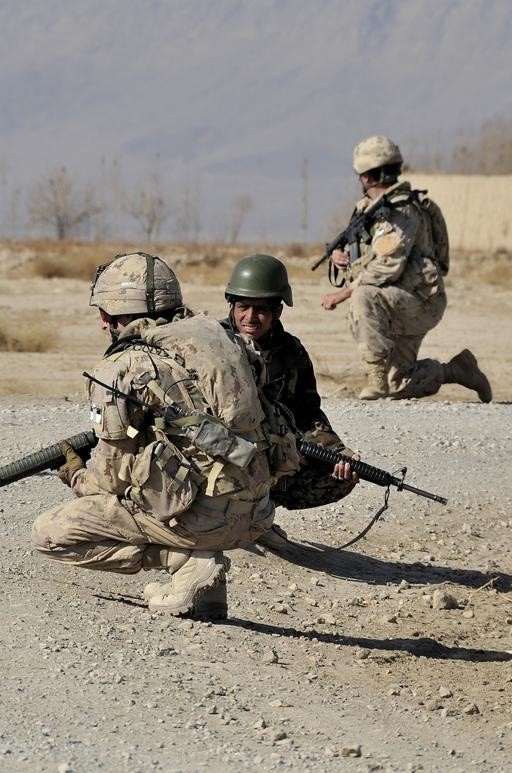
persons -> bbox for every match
[33,250,304,616]
[210,254,359,552]
[320,133,491,403]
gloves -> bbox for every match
[58,441,84,487]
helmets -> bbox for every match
[88,252,182,315]
[353,137,403,174]
[225,254,294,308]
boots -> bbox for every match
[144,547,231,616]
[359,365,389,399]
[443,349,492,403]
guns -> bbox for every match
[1,429,99,488]
[296,440,448,506]
[311,194,392,271]
